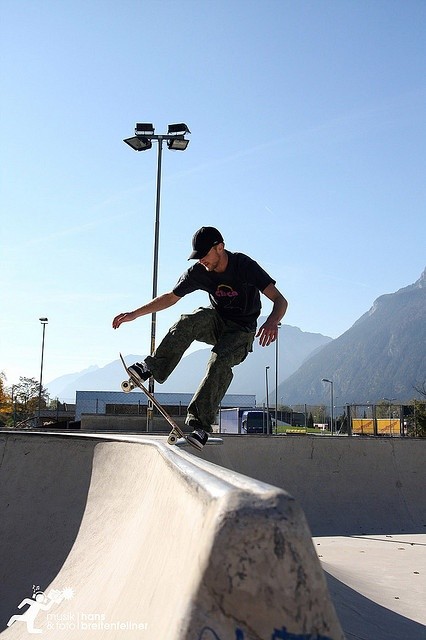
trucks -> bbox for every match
[218,408,275,434]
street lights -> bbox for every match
[265,366,270,435]
[37,317,48,427]
[122,122,192,432]
[320,378,333,436]
[382,398,397,437]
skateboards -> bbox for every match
[119,351,202,451]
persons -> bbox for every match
[111,226,289,450]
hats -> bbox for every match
[187,226,224,261]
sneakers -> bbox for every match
[187,429,208,449]
[128,362,153,383]
